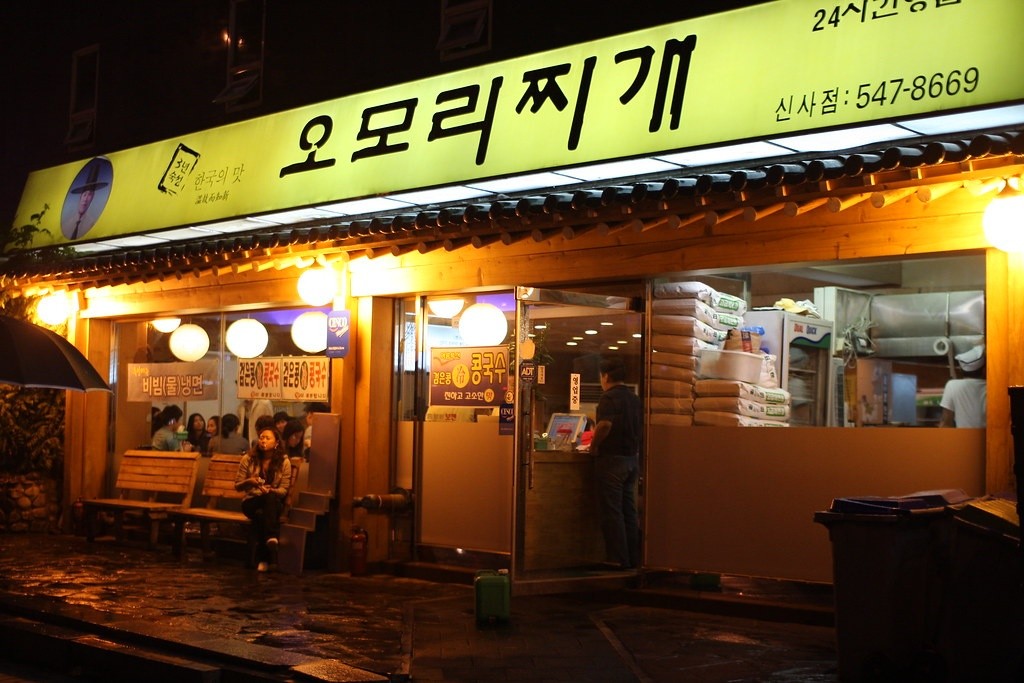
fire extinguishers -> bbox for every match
[351,526,368,574]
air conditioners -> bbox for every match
[572,383,638,423]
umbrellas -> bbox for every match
[0,315,116,397]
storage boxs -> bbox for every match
[842,357,892,426]
[813,286,874,354]
[698,347,765,385]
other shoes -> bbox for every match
[257,561,269,574]
[266,536,279,555]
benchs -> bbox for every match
[163,454,304,563]
[80,449,202,552]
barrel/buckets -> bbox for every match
[471,568,512,635]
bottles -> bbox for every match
[555,423,572,451]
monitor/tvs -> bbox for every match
[547,413,586,443]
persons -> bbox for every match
[234,426,292,574]
[151,379,331,462]
[587,360,643,573]
[581,418,595,446]
[936,344,987,428]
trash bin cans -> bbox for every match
[816,489,1024,683]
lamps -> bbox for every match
[151,317,181,334]
[167,317,210,362]
[459,303,507,346]
[37,294,72,327]
[982,176,1024,253]
[290,310,327,354]
[428,297,464,318]
[225,313,269,359]
[297,256,336,307]
